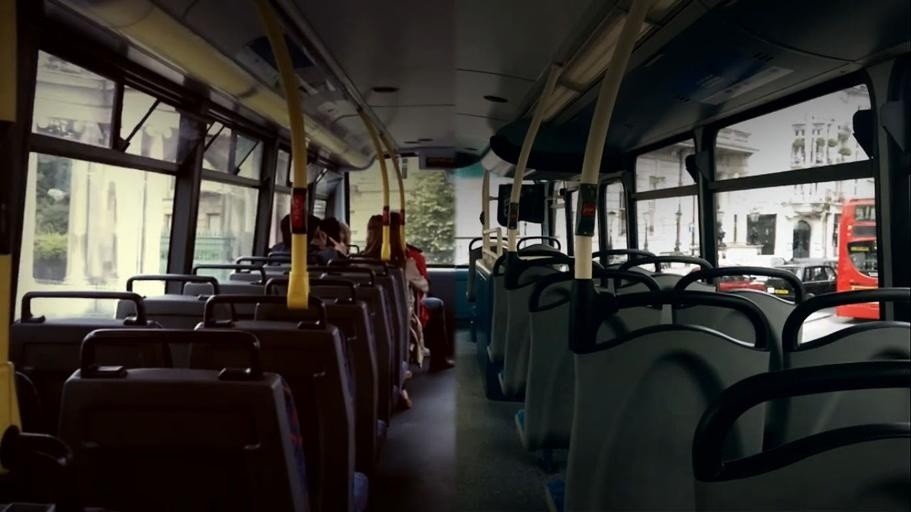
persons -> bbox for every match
[276,208,456,375]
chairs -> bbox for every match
[1,236,423,511]
[469,235,911,510]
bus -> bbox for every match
[836,196,880,320]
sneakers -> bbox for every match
[429,358,456,373]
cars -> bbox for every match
[767,264,837,301]
[690,268,766,291]
